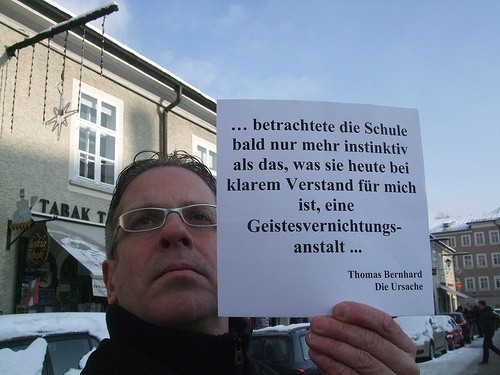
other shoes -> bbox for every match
[478,361,488,365]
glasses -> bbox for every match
[109,204,217,244]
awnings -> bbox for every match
[31,214,106,299]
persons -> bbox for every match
[472,298,500,374]
[71,149,423,375]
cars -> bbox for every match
[438,316,466,351]
[393,315,449,360]
[453,313,476,344]
[252,323,319,374]
[1,312,109,375]
